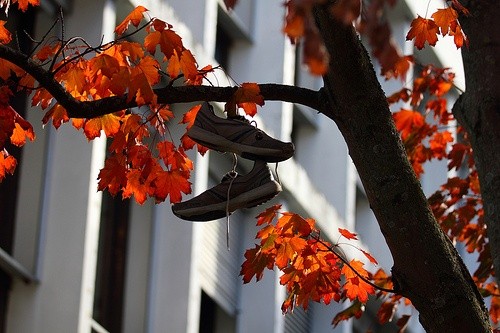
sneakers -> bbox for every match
[172,159,283,222]
[189,102,295,162]
